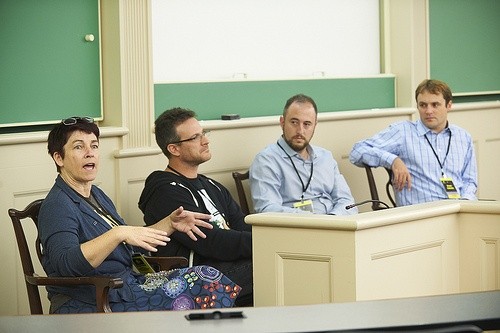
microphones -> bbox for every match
[345,199,389,210]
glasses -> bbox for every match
[166,130,211,150]
[54,117,94,137]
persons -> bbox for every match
[350,79,478,207]
[136,108,253,307]
[38,116,242,314]
[249,94,358,216]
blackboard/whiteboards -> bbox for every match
[0,0,104,128]
[426,0,500,97]
[152,1,397,131]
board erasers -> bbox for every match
[222,114,240,120]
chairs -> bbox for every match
[7,198,189,315]
[231,169,251,220]
[362,163,398,212]
